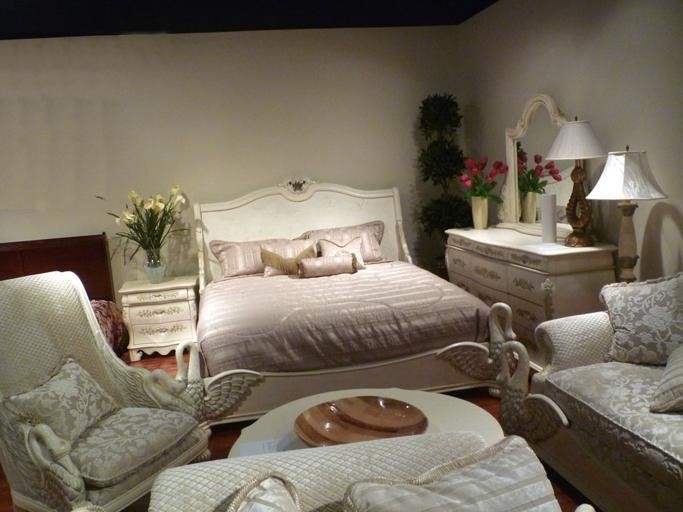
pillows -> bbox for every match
[218,472,299,512]
[345,434,563,511]
[648,343,683,413]
[3,354,121,448]
[599,270,683,366]
[210,219,394,280]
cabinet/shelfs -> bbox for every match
[444,228,619,372]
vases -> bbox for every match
[142,247,167,285]
[521,189,536,225]
[471,196,489,230]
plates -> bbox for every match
[292,395,427,449]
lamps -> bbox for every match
[545,115,610,248]
[585,144,668,283]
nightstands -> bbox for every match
[116,274,198,363]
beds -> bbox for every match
[174,175,517,432]
[0,231,129,353]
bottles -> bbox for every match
[540,194,556,244]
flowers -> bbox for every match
[516,141,562,191]
[95,183,192,268]
[460,156,510,204]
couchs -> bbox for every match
[495,268,683,512]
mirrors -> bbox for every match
[498,92,587,240]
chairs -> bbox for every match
[0,270,213,512]
[145,428,591,512]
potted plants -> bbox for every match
[418,92,469,280]
